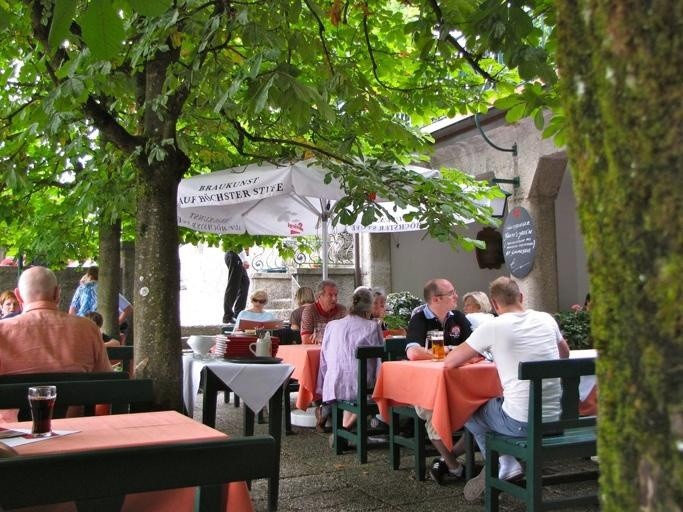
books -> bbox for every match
[215,333,280,358]
[239,317,284,331]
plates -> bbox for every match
[215,335,279,357]
[226,357,283,364]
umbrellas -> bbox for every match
[177,147,490,280]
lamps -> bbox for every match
[474,171,519,188]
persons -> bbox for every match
[222,247,250,323]
[0,264,133,424]
[231,290,277,334]
[444,277,570,501]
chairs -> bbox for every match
[0,324,600,512]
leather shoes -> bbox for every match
[314,403,326,433]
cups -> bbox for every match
[317,323,327,344]
[247,331,272,357]
[430,330,446,361]
[27,386,56,437]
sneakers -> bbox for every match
[429,456,522,501]
[222,315,236,324]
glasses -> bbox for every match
[250,297,266,304]
[430,288,457,298]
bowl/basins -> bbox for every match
[186,335,216,354]
